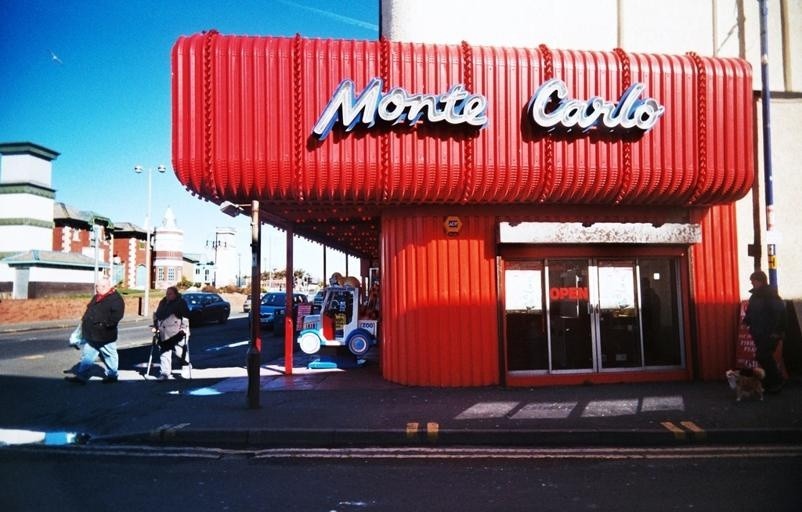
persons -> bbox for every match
[63,279,125,384]
[153,287,191,383]
[742,271,787,393]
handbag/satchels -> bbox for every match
[153,333,162,353]
[69,320,86,349]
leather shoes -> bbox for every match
[65,376,85,384]
[102,377,118,383]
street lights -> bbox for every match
[134,164,168,320]
[215,231,236,289]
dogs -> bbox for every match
[725,369,764,403]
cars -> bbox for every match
[182,292,230,324]
[244,294,263,313]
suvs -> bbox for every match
[249,292,309,332]
